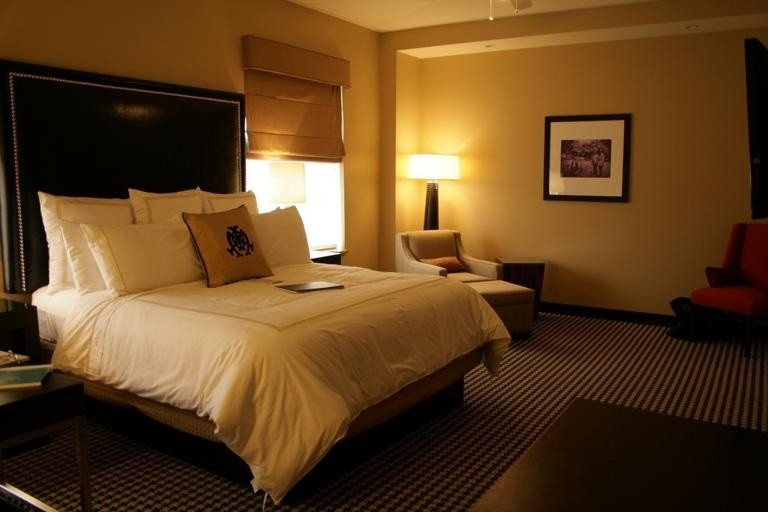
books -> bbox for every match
[0,364,55,390]
[274,281,345,295]
[0,349,31,366]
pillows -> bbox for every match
[179,201,274,288]
[37,190,137,297]
[704,266,743,288]
[128,186,203,227]
[249,206,311,268]
[59,215,105,293]
[80,217,206,297]
[420,255,465,275]
[201,190,259,216]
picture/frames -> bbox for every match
[544,115,631,204]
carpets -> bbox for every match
[467,395,768,512]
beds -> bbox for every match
[2,58,513,507]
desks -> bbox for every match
[0,352,92,512]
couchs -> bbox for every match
[691,222,768,358]
[395,229,534,341]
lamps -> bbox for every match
[406,154,461,231]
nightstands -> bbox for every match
[308,248,342,266]
[0,298,40,367]
[497,258,546,321]
